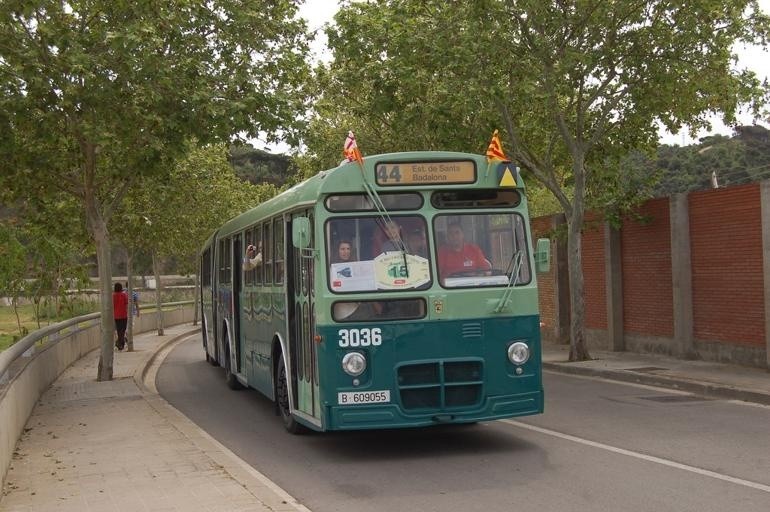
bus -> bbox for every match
[198,123,552,435]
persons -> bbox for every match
[379,218,408,255]
[405,228,425,256]
[331,238,355,262]
[112,282,129,350]
[437,221,492,287]
[242,238,262,272]
[122,281,140,344]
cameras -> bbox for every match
[248,245,257,250]
